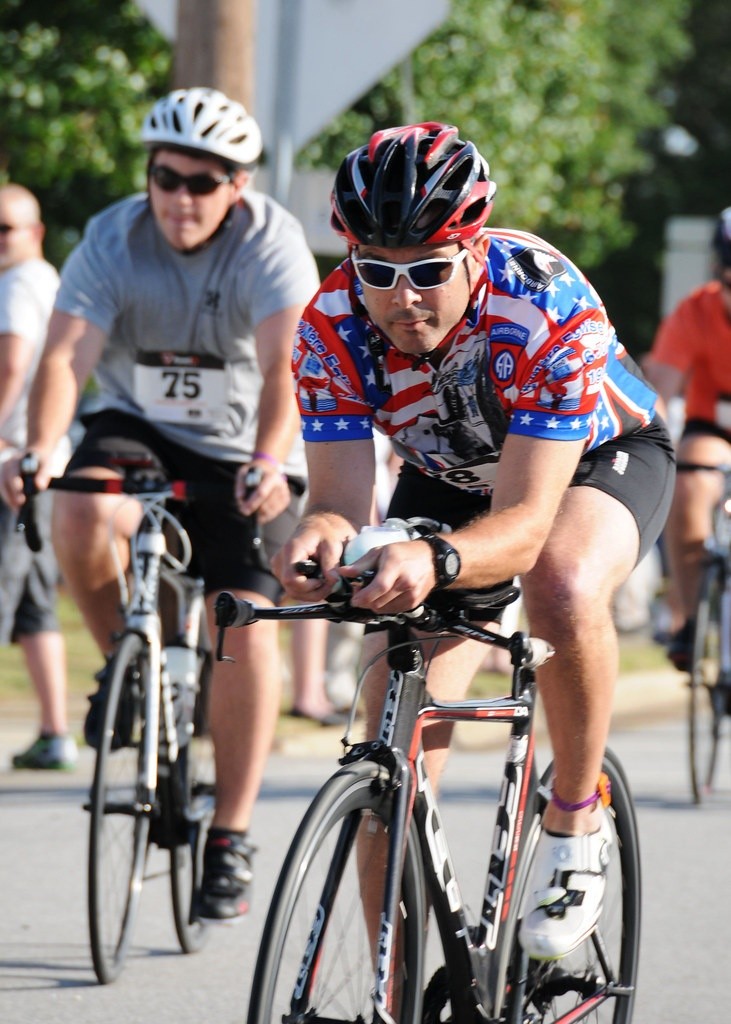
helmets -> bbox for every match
[143,88,265,172]
[330,123,497,249]
[713,206,731,265]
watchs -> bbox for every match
[413,534,461,588]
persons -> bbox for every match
[267,122,677,1024]
[0,184,88,772]
[636,205,731,713]
[2,87,404,918]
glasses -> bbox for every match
[150,165,232,198]
[351,245,467,291]
[0,223,35,235]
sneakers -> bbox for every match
[518,798,611,956]
[83,653,136,749]
[195,835,258,918]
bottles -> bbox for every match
[161,631,197,747]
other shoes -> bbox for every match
[718,672,731,717]
[12,738,79,769]
[670,616,695,670]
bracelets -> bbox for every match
[252,451,287,479]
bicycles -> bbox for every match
[638,409,731,806]
[209,512,646,1024]
[7,451,267,989]
